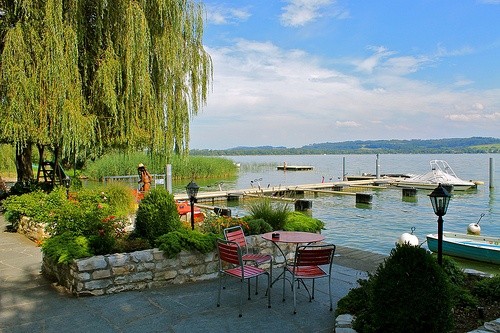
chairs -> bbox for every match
[215,237,271,318]
[191,213,207,224]
[222,226,273,295]
[177,204,187,221]
[283,244,336,314]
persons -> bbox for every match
[137,162,152,198]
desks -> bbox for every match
[261,231,325,302]
[177,206,201,221]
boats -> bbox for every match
[393,160,476,191]
[426,230,500,266]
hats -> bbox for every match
[138,163,144,167]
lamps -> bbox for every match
[427,183,452,217]
[185,180,199,203]
